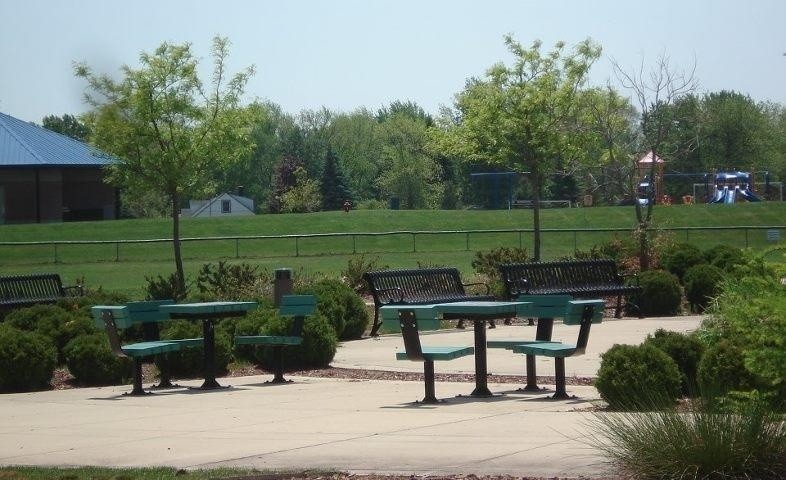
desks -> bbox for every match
[160,302,257,389]
[435,300,526,399]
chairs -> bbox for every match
[235,294,316,384]
[513,300,605,401]
[380,305,474,405]
[91,304,180,396]
[486,293,568,394]
[128,300,202,389]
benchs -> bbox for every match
[500,258,641,325]
[1,273,84,309]
[363,266,496,335]
